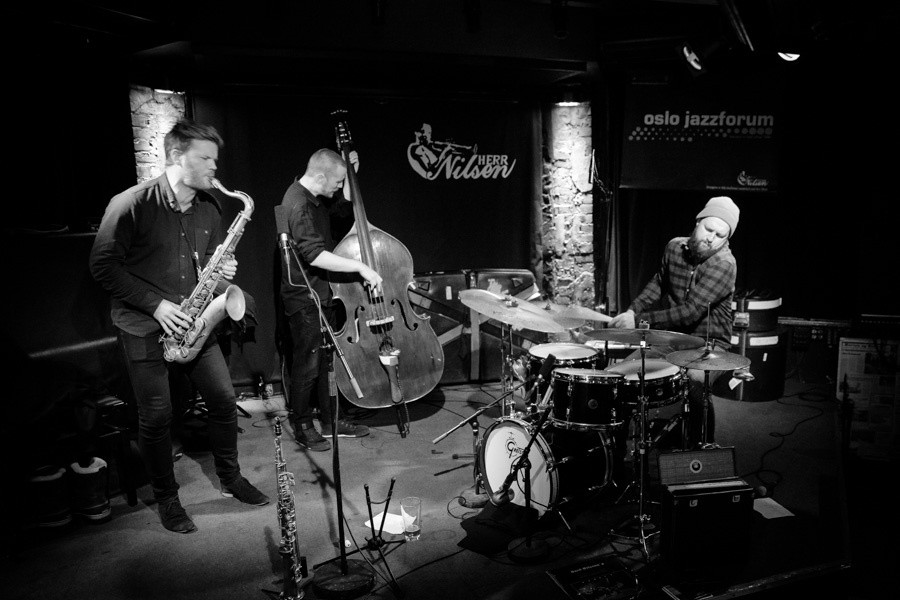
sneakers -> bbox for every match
[295,428,330,451]
[158,493,195,532]
[322,421,370,437]
[220,476,269,506]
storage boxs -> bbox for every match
[657,447,754,510]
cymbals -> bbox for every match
[666,349,751,371]
[458,288,564,334]
[583,328,706,347]
[530,301,585,331]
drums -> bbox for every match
[478,413,612,520]
[604,358,685,410]
[551,368,626,430]
[525,342,599,407]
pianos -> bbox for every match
[777,316,848,327]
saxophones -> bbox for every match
[273,416,305,600]
[158,181,255,364]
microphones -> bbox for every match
[531,354,556,391]
[280,232,291,265]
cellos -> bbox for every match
[327,109,446,409]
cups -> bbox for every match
[399,497,421,543]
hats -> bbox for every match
[696,196,741,238]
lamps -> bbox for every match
[681,35,721,79]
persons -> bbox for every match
[607,196,740,444]
[89,120,271,532]
[273,149,382,451]
